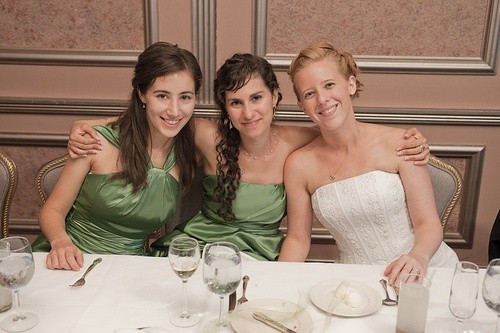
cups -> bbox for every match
[0,241,13,313]
[395,274,433,333]
[449,261,478,322]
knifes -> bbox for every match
[229,290,237,313]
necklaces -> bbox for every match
[321,147,349,181]
[238,129,277,160]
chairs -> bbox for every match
[0,153,17,240]
[426,154,463,231]
[36,154,69,205]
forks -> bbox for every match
[69,257,102,288]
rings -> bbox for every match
[412,267,420,274]
[420,145,424,150]
[74,141,79,147]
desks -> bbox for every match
[0,252,500,333]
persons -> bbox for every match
[278,44,460,291]
[66,53,432,262]
[20,41,204,257]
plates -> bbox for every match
[231,299,317,333]
[310,278,382,318]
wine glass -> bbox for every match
[481,259,500,333]
[0,236,39,332]
[167,236,201,328]
[201,241,242,333]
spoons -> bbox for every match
[379,279,398,306]
